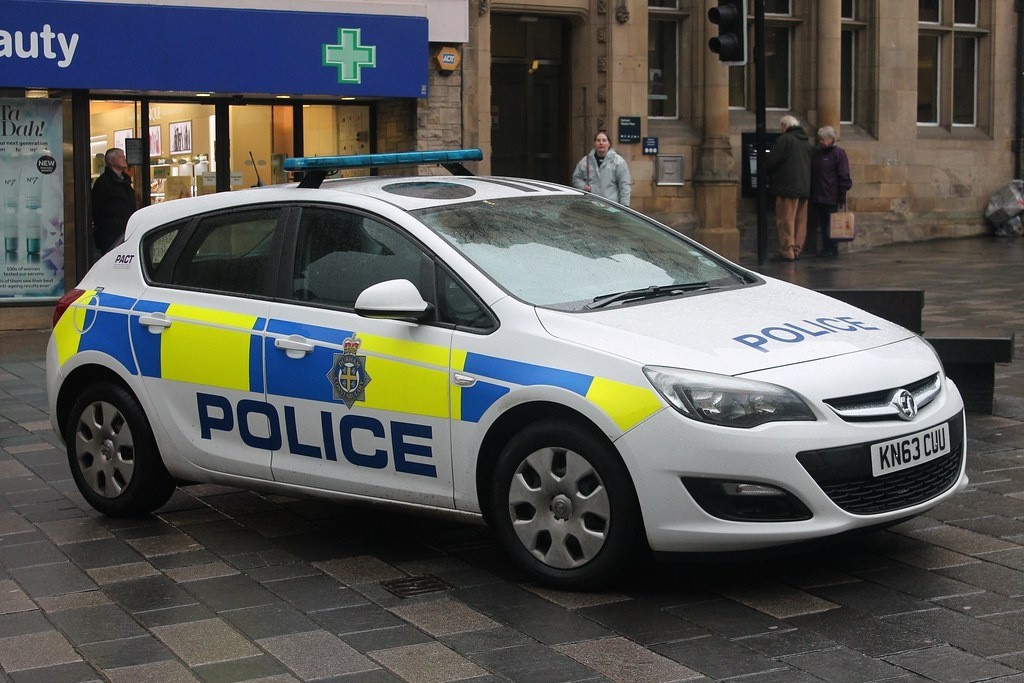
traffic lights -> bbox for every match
[709,0,748,67]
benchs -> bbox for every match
[815,287,1015,416]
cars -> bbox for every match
[47,146,970,587]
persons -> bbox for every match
[89,148,137,258]
[572,131,631,207]
[765,114,812,262]
[805,125,852,258]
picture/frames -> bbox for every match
[168,119,193,156]
[139,124,162,157]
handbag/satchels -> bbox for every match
[829,191,857,242]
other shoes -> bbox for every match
[770,254,795,262]
[821,247,838,257]
